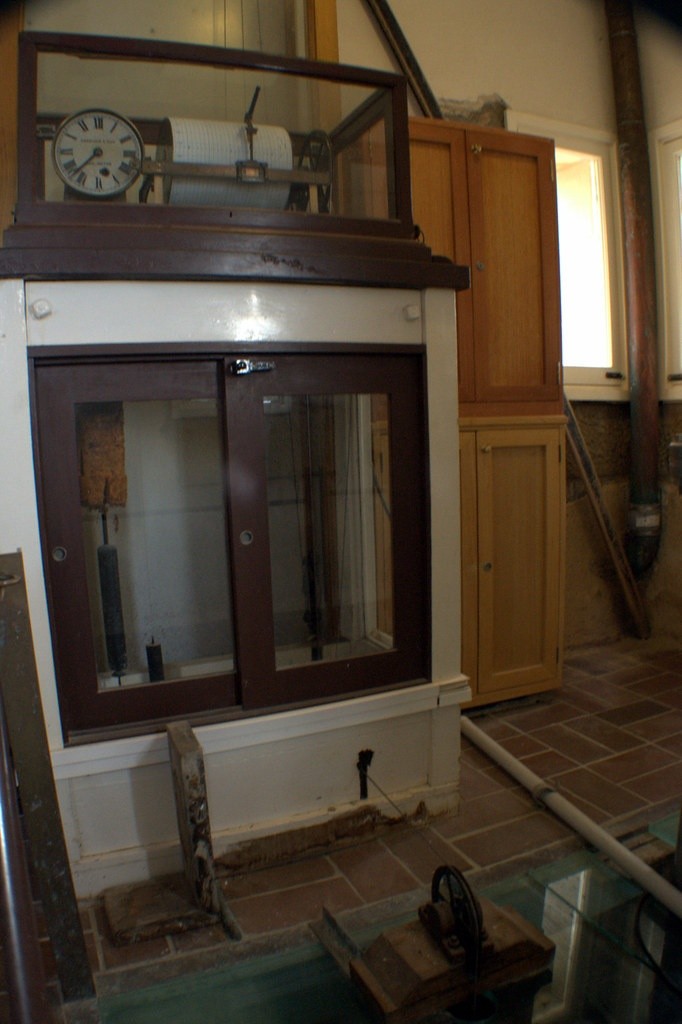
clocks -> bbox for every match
[50,107,146,204]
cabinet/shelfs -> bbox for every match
[331,115,564,713]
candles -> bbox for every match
[311,638,324,660]
[146,636,163,684]
